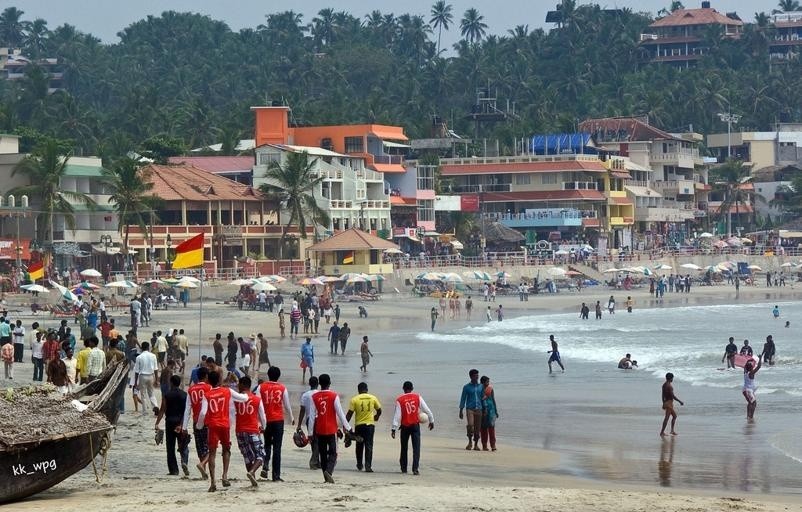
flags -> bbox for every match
[172,234,204,269]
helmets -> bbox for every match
[293,428,309,447]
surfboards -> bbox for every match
[603,302,614,309]
[733,354,755,369]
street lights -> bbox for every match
[470,229,482,258]
[214,233,230,269]
[286,232,298,277]
[98,233,114,285]
[716,104,743,157]
[167,233,174,269]
[415,226,427,246]
[627,223,638,259]
[29,238,41,254]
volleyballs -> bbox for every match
[419,413,428,423]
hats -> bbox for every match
[249,333,257,339]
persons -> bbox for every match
[548,334,565,373]
[721,335,776,419]
[618,353,637,369]
[1,259,436,491]
[132,220,275,225]
[658,373,684,436]
[457,368,500,451]
[383,209,802,332]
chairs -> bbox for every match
[0,277,381,319]
[414,269,761,299]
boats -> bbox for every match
[0,348,134,505]
